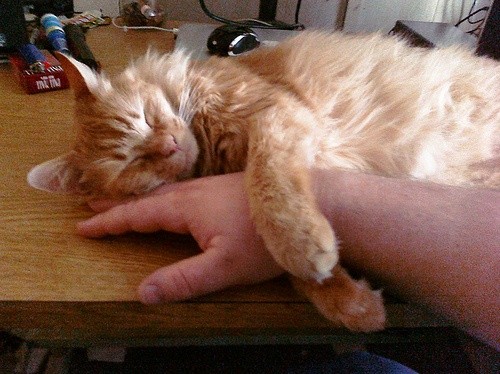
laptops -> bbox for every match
[174,23,303,59]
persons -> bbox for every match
[74,167,500,374]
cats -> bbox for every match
[26,29,499,333]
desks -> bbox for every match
[0,16,456,374]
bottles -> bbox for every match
[118,0,167,28]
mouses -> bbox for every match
[207,25,260,57]
